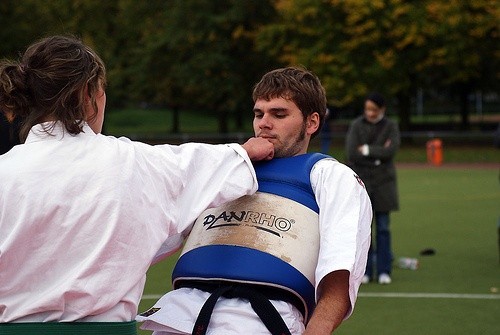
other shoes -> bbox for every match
[362,274,370,284]
[379,272,392,283]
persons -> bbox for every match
[0,34,275,335]
[345,91,400,283]
[136,65,373,335]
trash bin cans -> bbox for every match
[426,140,442,166]
